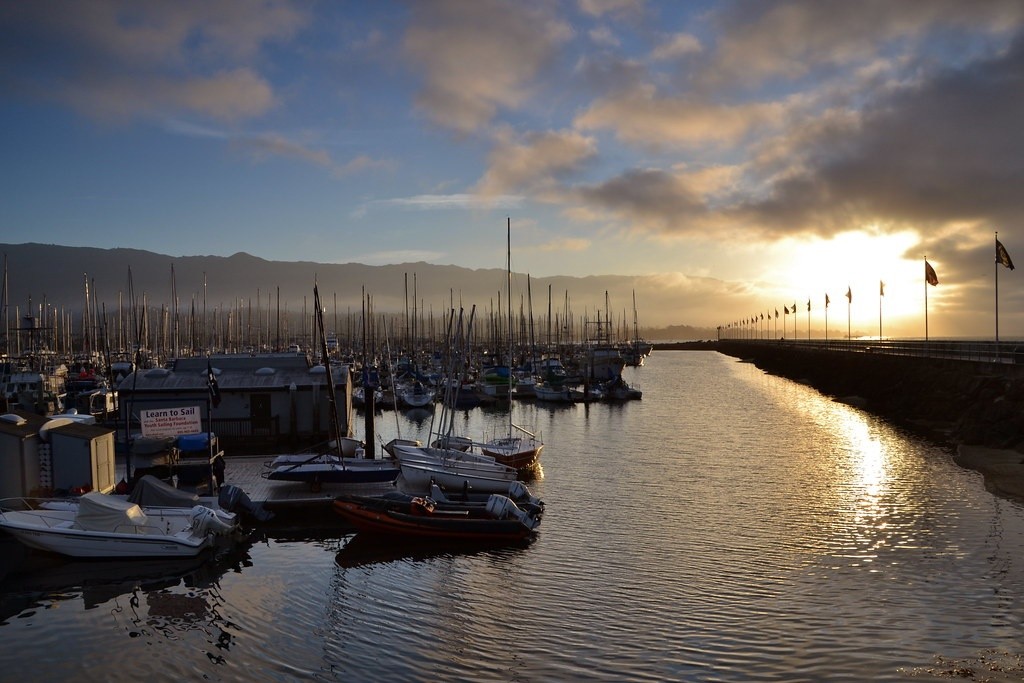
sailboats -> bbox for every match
[0,263,652,489]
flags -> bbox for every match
[996,239,1015,271]
[734,280,885,326]
[925,262,939,287]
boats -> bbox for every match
[0,475,246,558]
[332,481,545,544]
